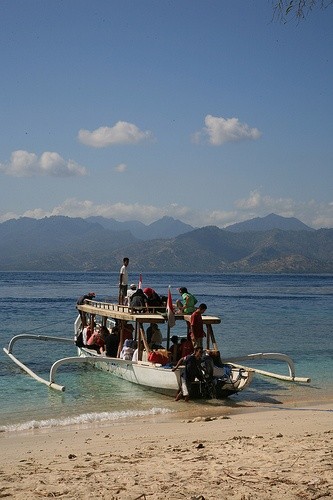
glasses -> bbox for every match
[197,350,203,353]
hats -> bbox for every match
[89,292,95,297]
[144,288,152,293]
[130,284,136,288]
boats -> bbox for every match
[4,298,310,402]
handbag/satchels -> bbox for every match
[75,332,84,347]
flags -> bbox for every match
[166,291,175,327]
[138,275,142,290]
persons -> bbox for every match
[176,287,198,314]
[132,323,194,364]
[119,258,129,301]
[76,322,135,357]
[127,284,164,312]
[75,292,95,327]
[189,303,208,352]
[172,346,203,403]
